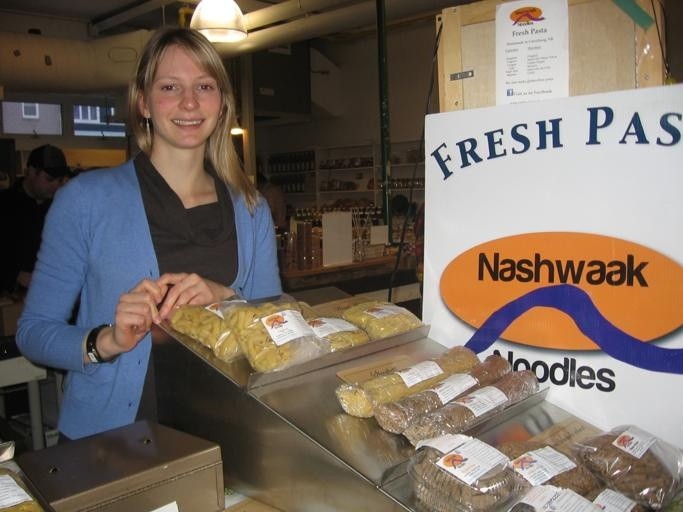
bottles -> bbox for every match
[391,178,423,189]
[257,159,313,171]
[291,205,382,227]
[279,177,304,192]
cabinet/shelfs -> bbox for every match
[267,140,425,307]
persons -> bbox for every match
[381,194,414,242]
[1,142,68,302]
[13,27,284,445]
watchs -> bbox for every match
[84,323,117,365]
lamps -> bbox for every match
[230,119,243,135]
[190,1,248,43]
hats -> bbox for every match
[29,144,74,178]
